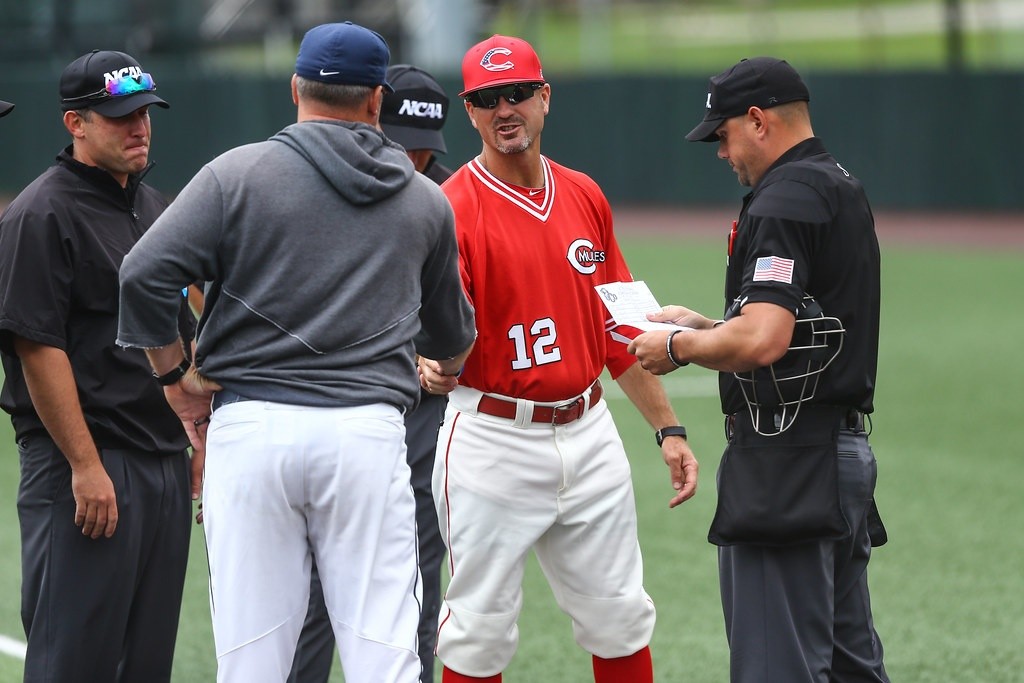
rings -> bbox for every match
[194,416,210,426]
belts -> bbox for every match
[477,379,604,427]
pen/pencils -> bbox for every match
[729,221,737,257]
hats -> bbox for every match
[61,48,171,118]
[0,100,16,118]
[685,58,808,142]
[461,33,545,95]
[296,20,390,88]
[378,64,450,156]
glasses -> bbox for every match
[464,83,542,108]
[87,73,156,100]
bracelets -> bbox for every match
[666,330,690,368]
[655,426,686,448]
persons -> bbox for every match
[116,20,477,683]
[0,50,207,682]
[286,66,457,683]
[417,35,698,683]
[627,56,890,683]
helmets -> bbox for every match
[725,291,844,437]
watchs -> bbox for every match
[152,357,191,386]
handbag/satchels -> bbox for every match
[709,426,847,548]
